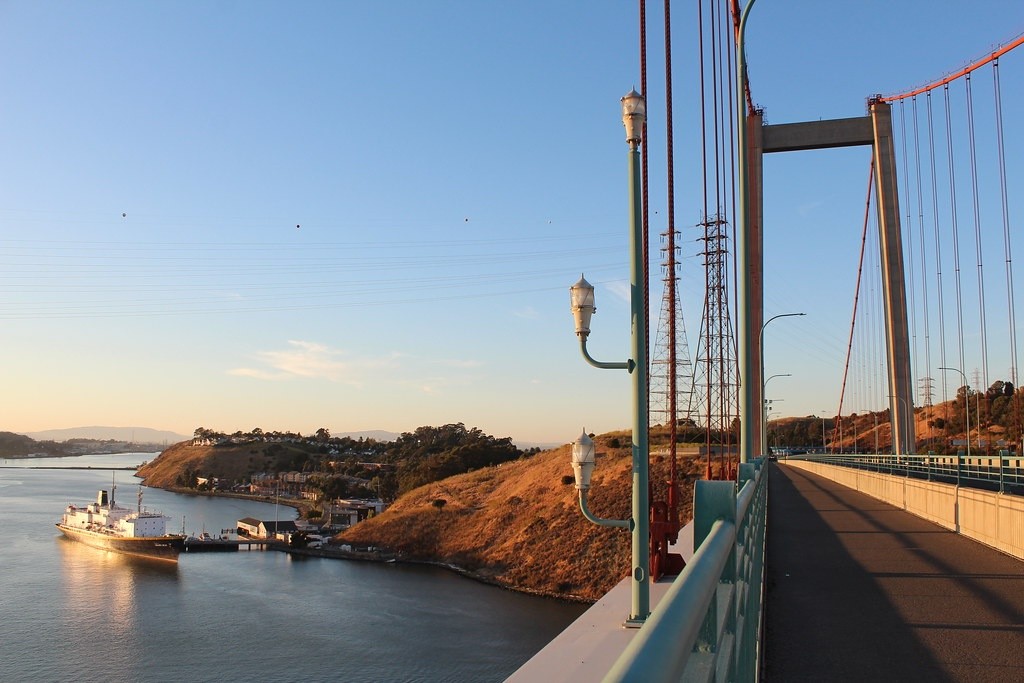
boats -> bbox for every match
[54,470,188,563]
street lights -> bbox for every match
[569,84,662,628]
[817,367,972,458]
[757,310,807,456]
